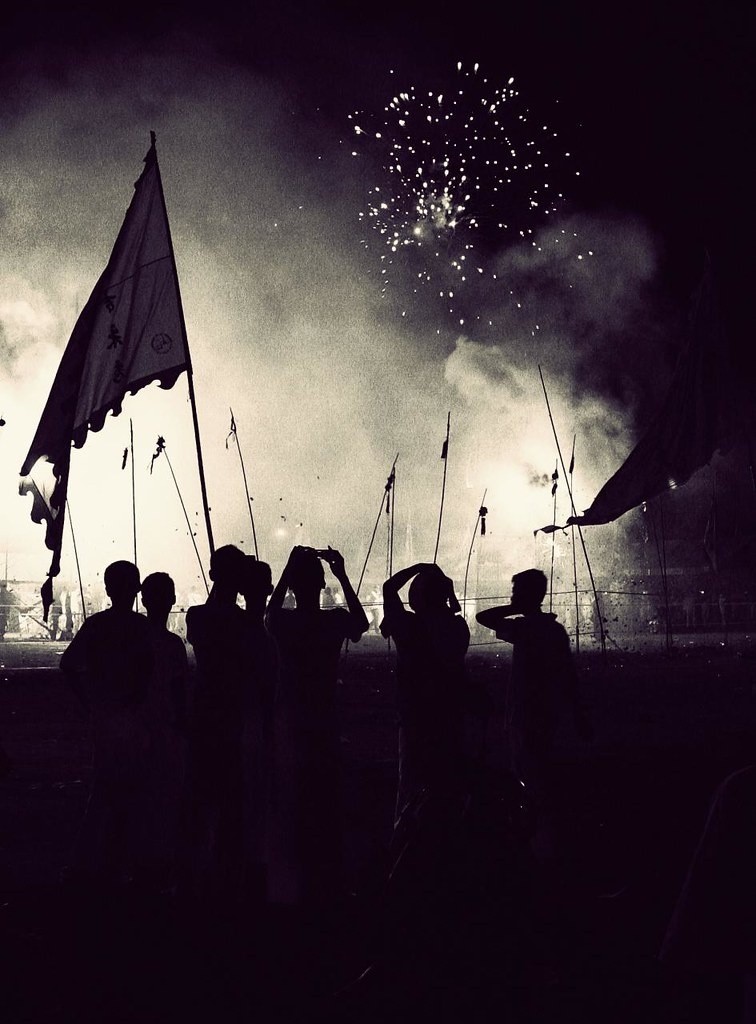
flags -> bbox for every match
[20,147,191,624]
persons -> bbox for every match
[58,544,607,710]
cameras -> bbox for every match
[315,550,334,561]
